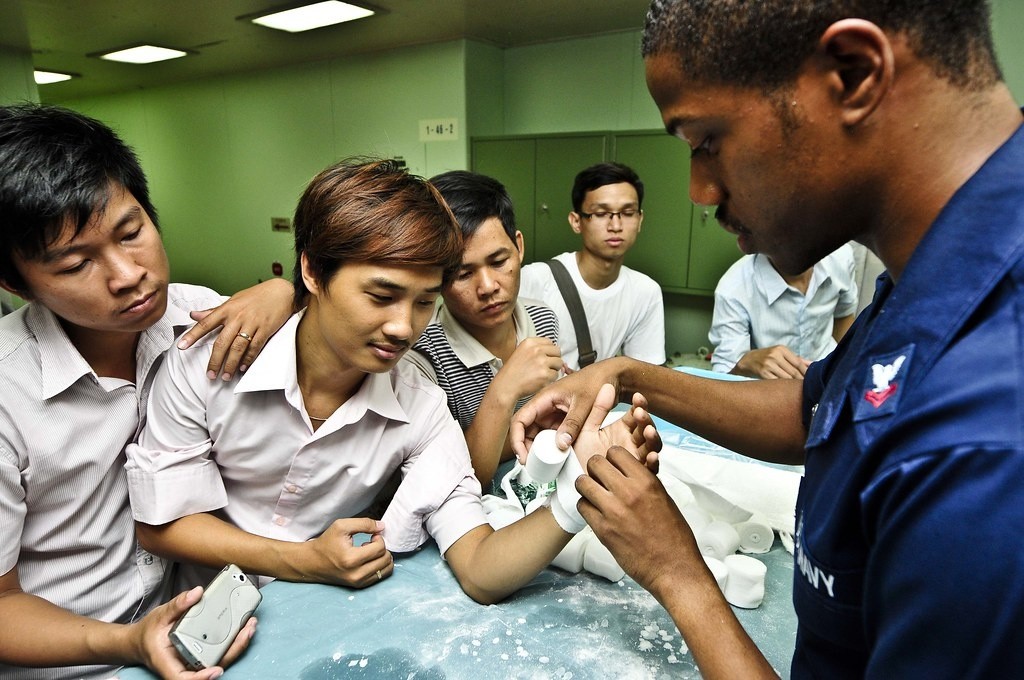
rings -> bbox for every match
[376,568,383,579]
[237,332,249,338]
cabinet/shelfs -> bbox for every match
[607,126,746,298]
[470,128,611,269]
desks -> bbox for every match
[113,366,802,677]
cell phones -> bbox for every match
[168,564,263,670]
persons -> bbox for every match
[707,241,859,380]
[0,104,294,680]
[123,156,664,606]
[517,161,666,378]
[412,170,564,488]
[510,0,1024,680]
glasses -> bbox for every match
[577,208,643,222]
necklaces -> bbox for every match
[310,416,328,422]
